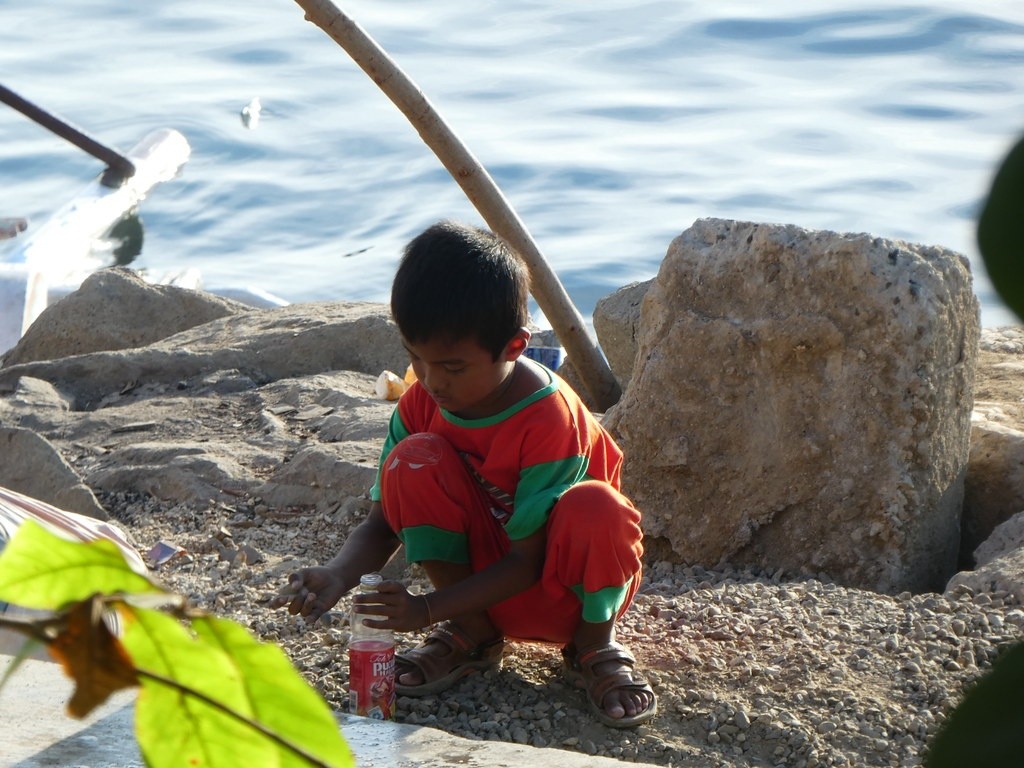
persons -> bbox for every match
[269,216,659,729]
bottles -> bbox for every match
[346,574,396,724]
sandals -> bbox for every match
[392,620,505,695]
[561,638,656,727]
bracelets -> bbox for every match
[416,593,434,640]
